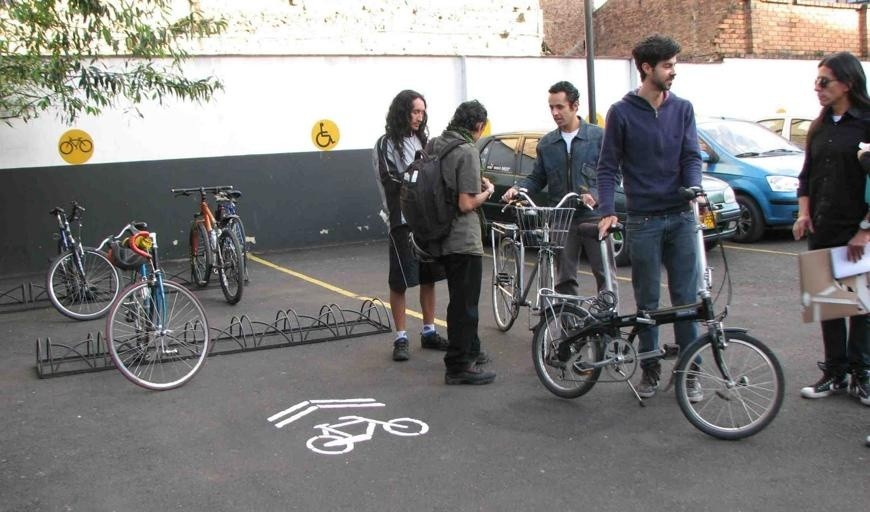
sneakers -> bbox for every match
[849,367,870,406]
[420,330,450,351]
[392,337,410,361]
[800,362,848,399]
[637,374,659,398]
[470,350,489,369]
[686,380,703,403]
[445,366,496,385]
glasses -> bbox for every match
[814,78,839,88]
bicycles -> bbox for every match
[525,183,787,442]
[213,186,250,281]
[43,197,125,325]
[167,181,246,305]
[486,185,601,363]
[102,216,211,395]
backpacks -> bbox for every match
[399,136,466,242]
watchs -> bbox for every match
[486,190,493,200]
[859,220,870,230]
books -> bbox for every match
[830,242,870,279]
[857,141,870,173]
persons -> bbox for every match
[424,99,497,385]
[791,51,870,402]
[598,34,708,402]
[501,81,621,340]
[372,90,450,360]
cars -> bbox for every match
[470,127,742,269]
[753,112,823,148]
[690,114,810,249]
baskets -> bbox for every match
[515,205,576,250]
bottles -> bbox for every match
[207,229,217,250]
